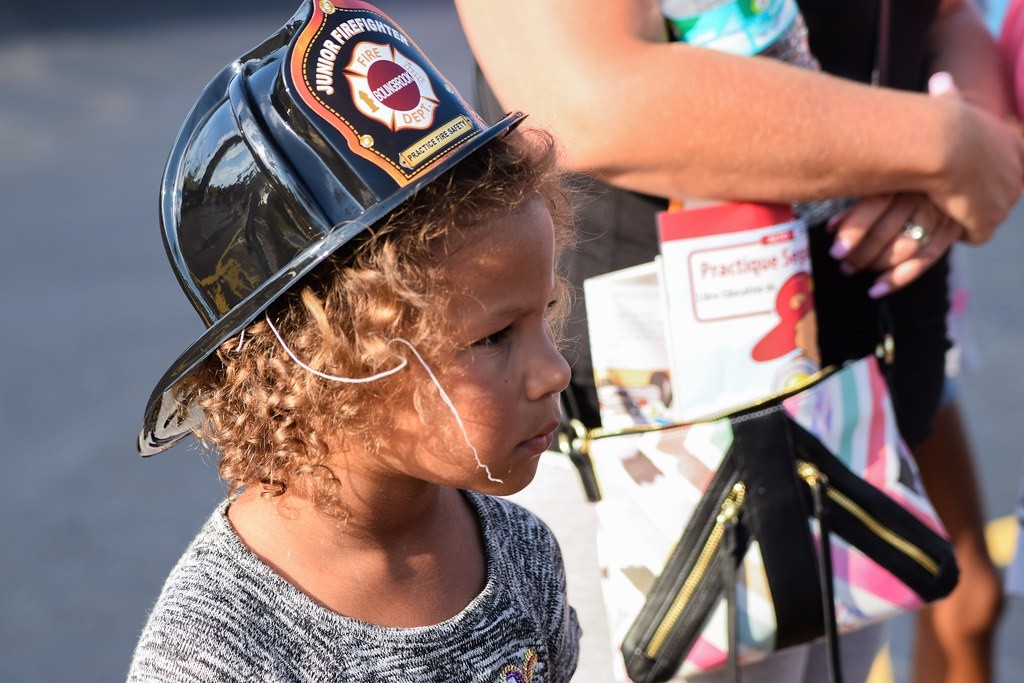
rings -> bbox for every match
[904,225,931,247]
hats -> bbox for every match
[137,0,528,457]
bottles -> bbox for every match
[664,0,859,227]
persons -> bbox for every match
[124,0,580,683]
[455,0,1024,683]
